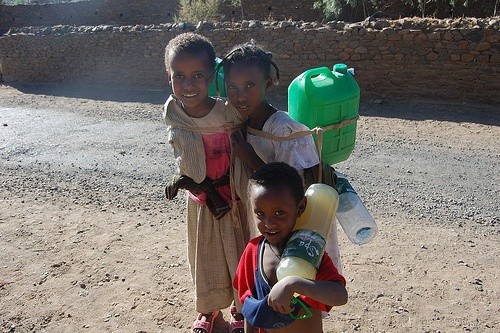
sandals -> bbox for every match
[230,300,244,333]
[191,309,219,333]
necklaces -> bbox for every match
[268,244,281,260]
[260,102,273,130]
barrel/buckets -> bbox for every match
[207,57,226,97]
[288,64,360,165]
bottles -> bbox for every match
[276,183,339,320]
[329,167,378,244]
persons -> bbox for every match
[162,32,256,333]
[231,160,348,333]
[205,43,343,318]
